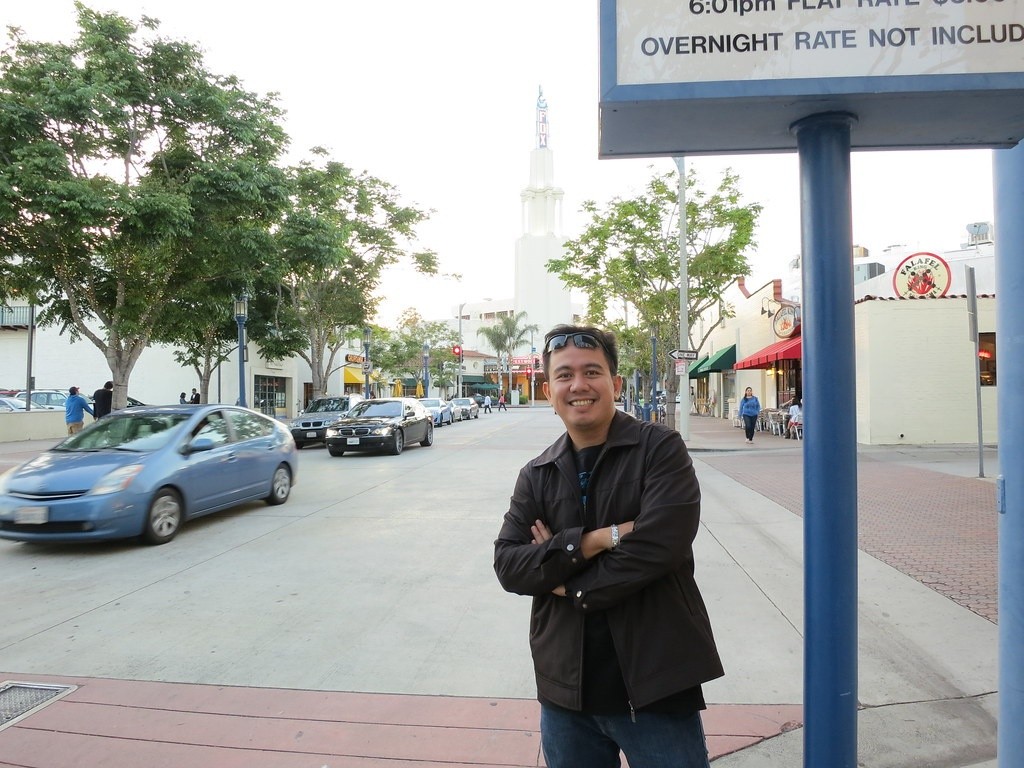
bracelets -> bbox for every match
[611,524,619,547]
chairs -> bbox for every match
[733,409,802,441]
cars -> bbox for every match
[0,404,299,547]
[0,387,151,412]
[443,401,463,423]
[450,398,479,421]
[325,396,435,457]
[415,397,452,427]
[648,391,681,404]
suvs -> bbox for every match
[289,393,365,448]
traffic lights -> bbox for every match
[457,349,464,364]
[535,358,540,369]
[454,345,461,354]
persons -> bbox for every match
[739,387,760,443]
[345,391,349,395]
[93,381,113,421]
[782,395,802,439]
[362,387,370,399]
[498,394,507,411]
[180,388,200,404]
[484,394,492,413]
[369,390,376,399]
[65,387,94,436]
[493,324,725,768]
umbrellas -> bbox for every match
[416,380,424,397]
[392,378,403,397]
[471,383,498,396]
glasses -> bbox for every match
[544,333,611,357]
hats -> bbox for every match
[69,387,79,393]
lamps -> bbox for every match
[793,306,801,327]
[761,297,771,315]
[767,299,779,318]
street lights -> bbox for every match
[458,297,493,398]
[363,325,372,400]
[233,291,247,408]
[651,320,659,412]
[423,342,430,398]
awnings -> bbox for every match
[698,344,736,373]
[457,375,484,382]
[344,366,376,383]
[689,356,709,379]
[393,379,425,386]
[733,336,802,370]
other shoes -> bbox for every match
[745,437,754,444]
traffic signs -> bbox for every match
[668,348,699,362]
